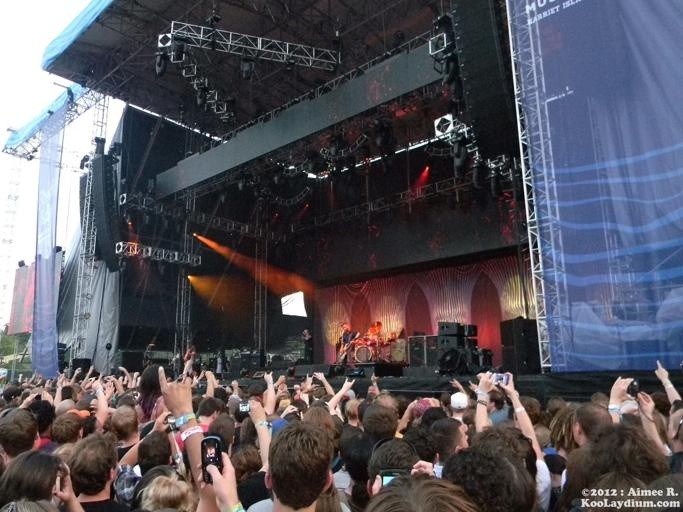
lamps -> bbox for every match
[149,15,507,200]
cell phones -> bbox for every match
[201,436,222,484]
[381,469,404,486]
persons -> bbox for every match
[0,360,681,510]
[181,340,197,380]
[301,329,314,364]
[338,322,360,364]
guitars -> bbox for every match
[336,331,360,364]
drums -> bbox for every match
[354,345,375,362]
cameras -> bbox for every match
[492,374,508,384]
[166,415,175,424]
[239,402,249,417]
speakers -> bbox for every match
[500,318,540,375]
[438,321,478,369]
[450,1,520,163]
[92,155,120,272]
[375,363,402,376]
[72,358,91,379]
[295,364,333,377]
[120,350,143,371]
